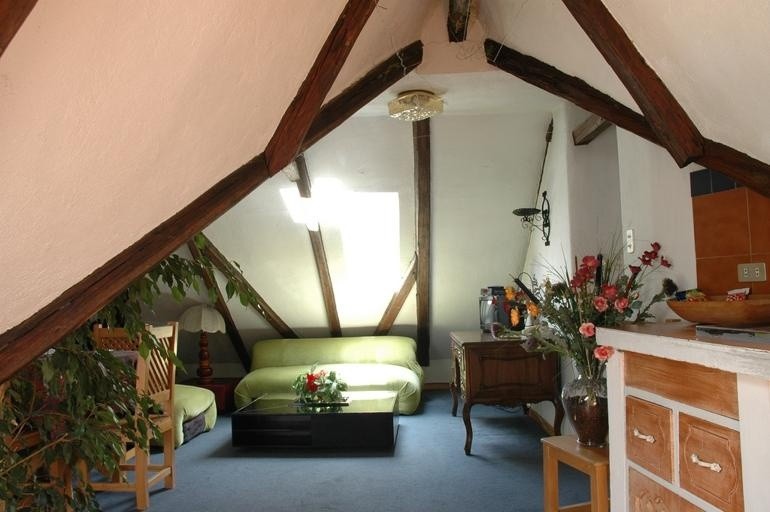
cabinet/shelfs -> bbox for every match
[591,321,770,512]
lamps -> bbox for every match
[178,303,228,384]
[386,89,449,124]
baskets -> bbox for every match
[664,292,770,328]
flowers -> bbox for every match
[489,213,680,405]
[287,357,350,406]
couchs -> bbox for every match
[234,334,426,416]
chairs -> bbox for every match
[1,319,179,512]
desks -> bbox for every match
[230,390,402,457]
[447,329,567,457]
[539,434,611,511]
[180,376,245,416]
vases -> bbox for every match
[559,363,609,449]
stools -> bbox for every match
[150,380,217,450]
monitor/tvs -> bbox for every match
[478,297,527,332]
